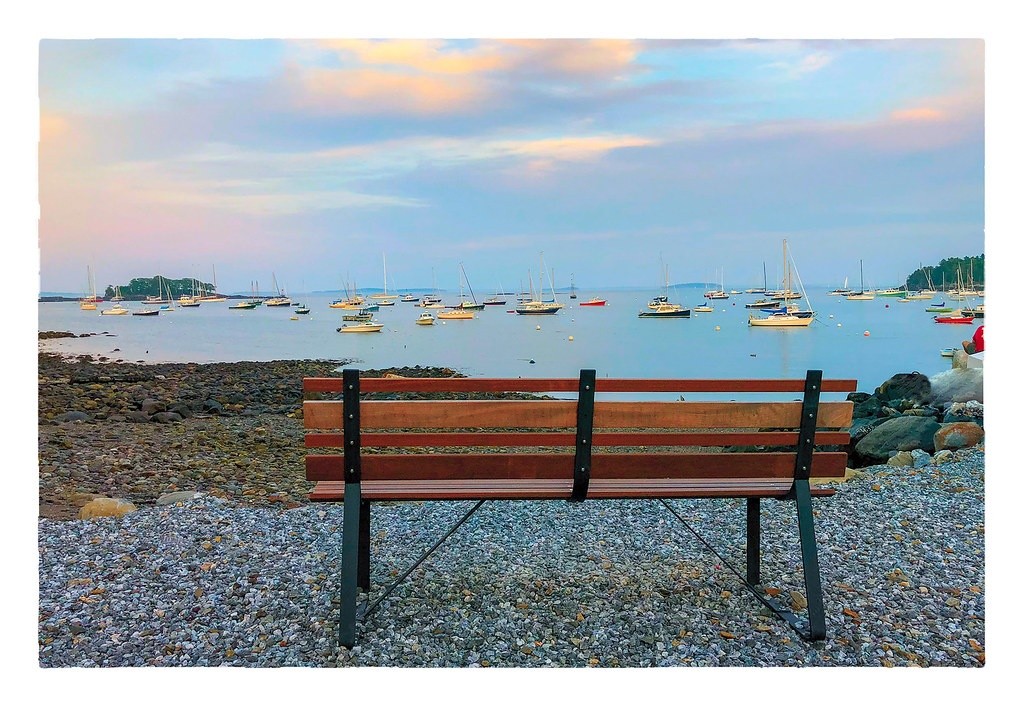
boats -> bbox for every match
[579,296,608,306]
[335,320,383,332]
[415,309,434,325]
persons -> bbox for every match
[961,325,984,355]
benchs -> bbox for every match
[299,368,859,652]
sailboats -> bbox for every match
[482,276,507,306]
[228,272,310,320]
[570,274,577,299]
[506,249,562,316]
[329,253,485,321]
[636,248,817,328]
[79,264,227,316]
[826,259,984,324]
